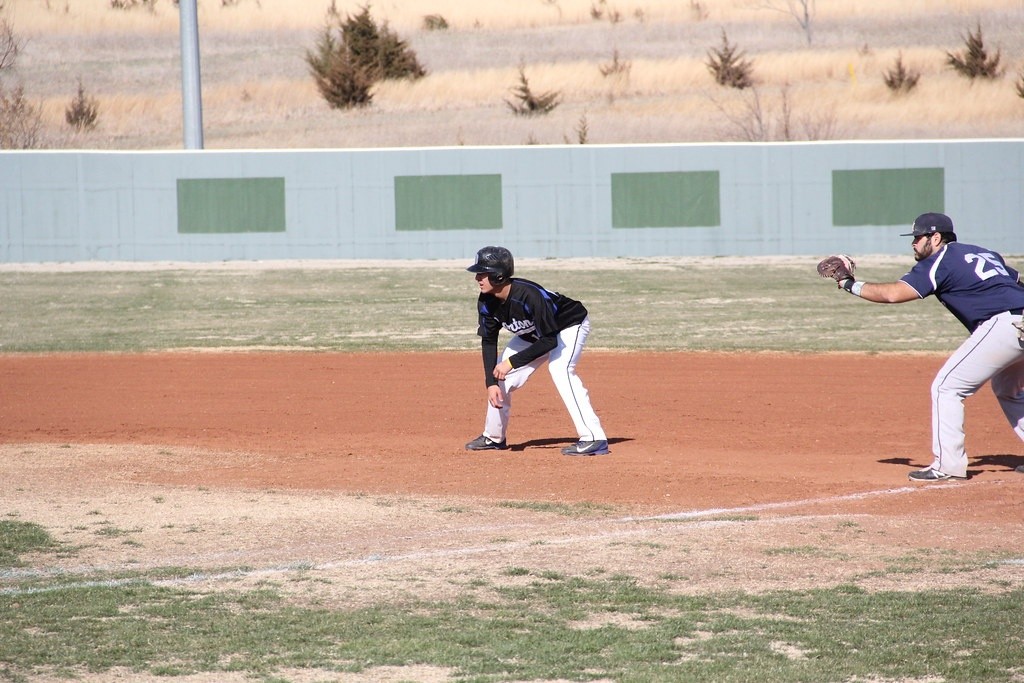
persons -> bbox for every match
[838,212,1024,483]
[465,246,609,456]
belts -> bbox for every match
[1010,308,1024,315]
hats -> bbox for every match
[899,213,953,236]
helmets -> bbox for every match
[467,246,514,286]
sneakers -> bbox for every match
[561,441,609,456]
[908,465,967,482]
[465,435,506,450]
[1015,465,1024,473]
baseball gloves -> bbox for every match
[816,254,856,290]
